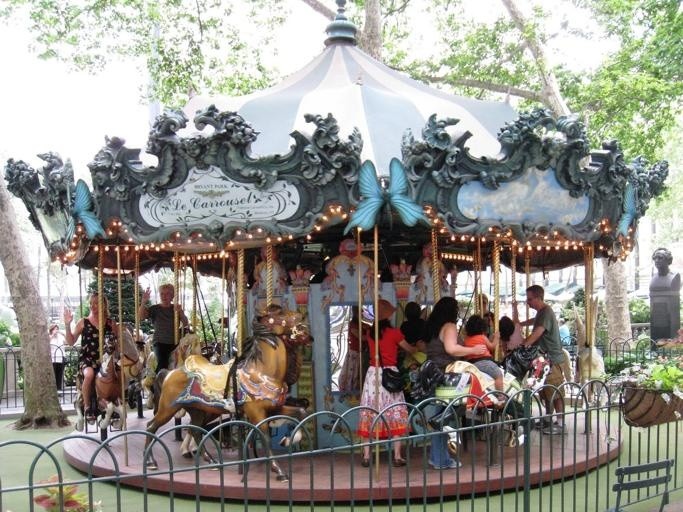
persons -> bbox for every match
[649,248,680,350]
[139,284,187,409]
[64,294,121,420]
[359,294,523,470]
[49,325,66,397]
[520,285,567,435]
[339,306,371,391]
[558,318,571,346]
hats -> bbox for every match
[374,299,397,320]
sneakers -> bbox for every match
[428,458,462,470]
[535,420,552,428]
[542,421,568,435]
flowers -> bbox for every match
[609,356,683,420]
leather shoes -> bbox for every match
[361,458,370,466]
[393,459,407,467]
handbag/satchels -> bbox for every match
[381,368,406,392]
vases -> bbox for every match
[622,387,683,429]
